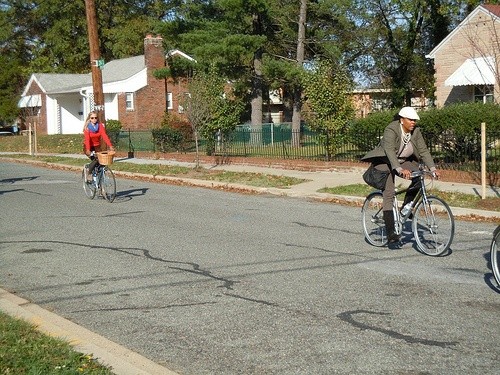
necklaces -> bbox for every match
[400,123,411,145]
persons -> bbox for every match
[82,111,115,185]
[361,107,441,250]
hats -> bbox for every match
[398,106,420,120]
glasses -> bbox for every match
[91,116,98,119]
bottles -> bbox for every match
[400,201,414,215]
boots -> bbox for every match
[400,188,419,220]
[382,210,400,244]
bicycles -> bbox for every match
[82,150,116,203]
[490,223,500,289]
[360,163,455,257]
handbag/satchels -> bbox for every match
[362,166,391,190]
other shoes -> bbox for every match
[87,174,93,184]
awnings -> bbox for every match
[17,94,42,109]
[445,57,496,86]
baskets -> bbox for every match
[96,151,115,166]
